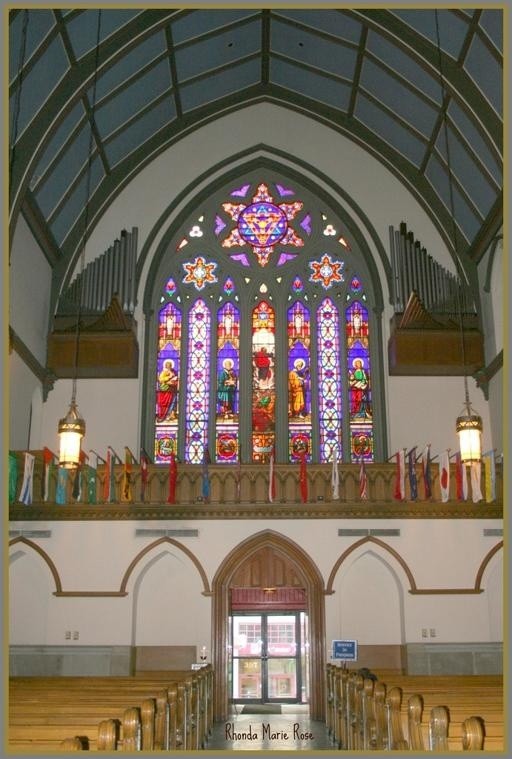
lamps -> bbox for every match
[58,21,102,471]
[433,20,483,466]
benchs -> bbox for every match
[5,670,212,754]
[324,668,504,754]
